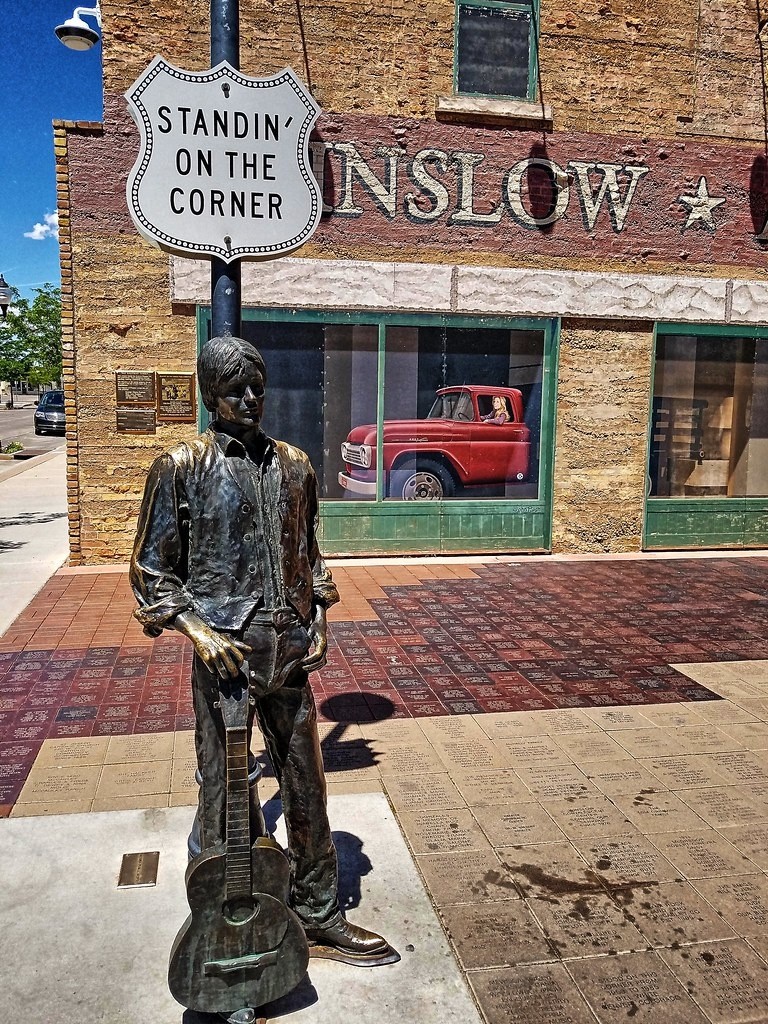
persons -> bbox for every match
[130,336,389,960]
[480,396,510,425]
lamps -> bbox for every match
[707,397,733,459]
[53,0,102,51]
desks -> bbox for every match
[674,457,731,499]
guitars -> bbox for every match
[166,658,310,1014]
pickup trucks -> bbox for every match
[337,384,534,501]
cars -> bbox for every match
[33,389,66,435]
